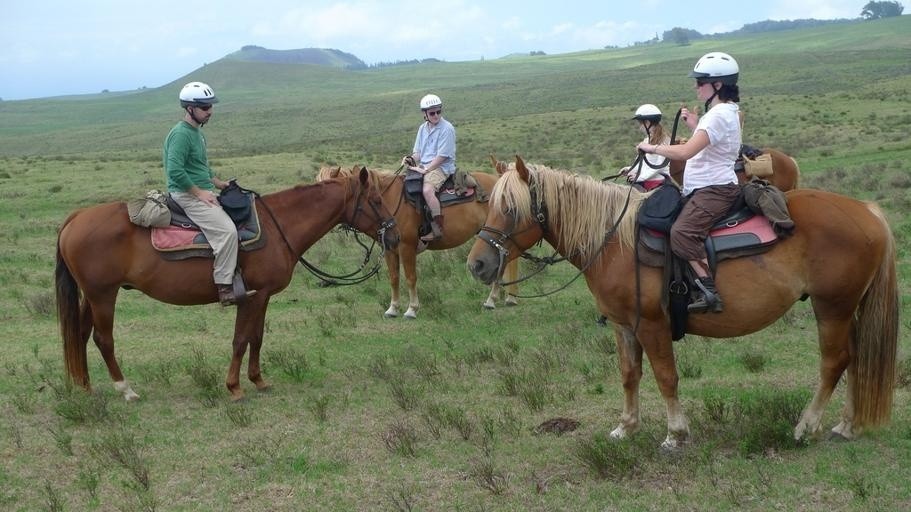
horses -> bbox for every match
[467,154,898,453]
[316,164,519,320]
[56,164,401,403]
[597,148,799,328]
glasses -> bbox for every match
[427,110,441,116]
[197,104,212,110]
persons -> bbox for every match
[164,81,259,306]
[616,52,742,314]
[401,94,456,241]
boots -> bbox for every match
[687,277,723,313]
[420,215,444,242]
[216,284,258,306]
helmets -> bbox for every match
[687,52,739,78]
[420,94,442,110]
[631,104,661,120]
[179,82,219,103]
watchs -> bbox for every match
[424,167,430,172]
